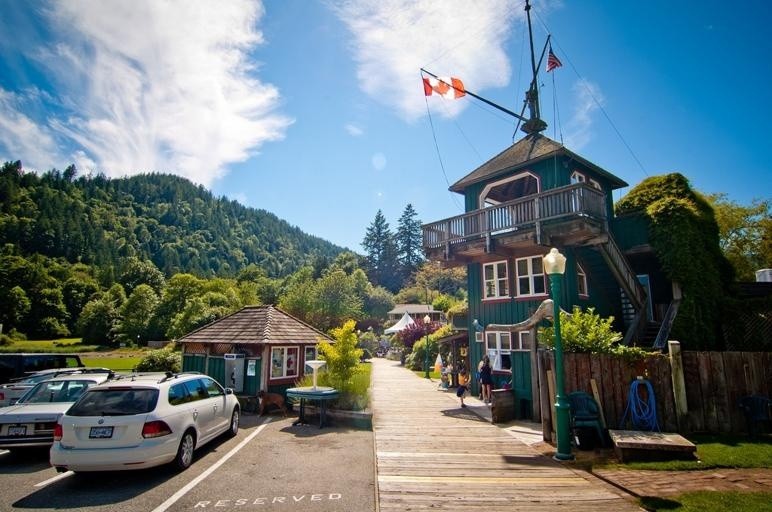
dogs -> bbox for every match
[255,390,288,417]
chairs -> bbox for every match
[567,391,603,450]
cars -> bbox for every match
[0,373,122,455]
[50,372,241,471]
[0,367,113,407]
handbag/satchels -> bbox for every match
[475,371,481,382]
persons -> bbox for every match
[479,355,494,403]
[457,368,471,408]
[475,361,484,400]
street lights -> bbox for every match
[424,315,430,378]
[542,247,574,461]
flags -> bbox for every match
[432,80,451,96]
[422,78,433,97]
[450,78,465,99]
[546,46,562,73]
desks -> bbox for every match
[287,387,339,429]
[296,360,334,392]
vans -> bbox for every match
[0,354,85,383]
